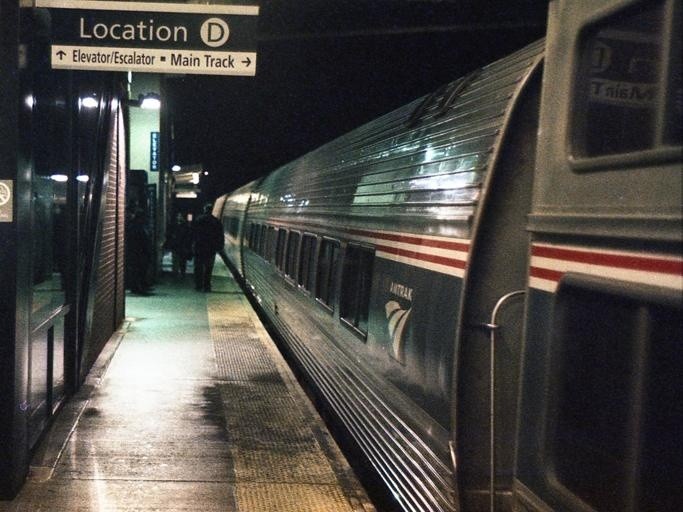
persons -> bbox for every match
[125,195,225,292]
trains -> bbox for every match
[208,0,683,512]
